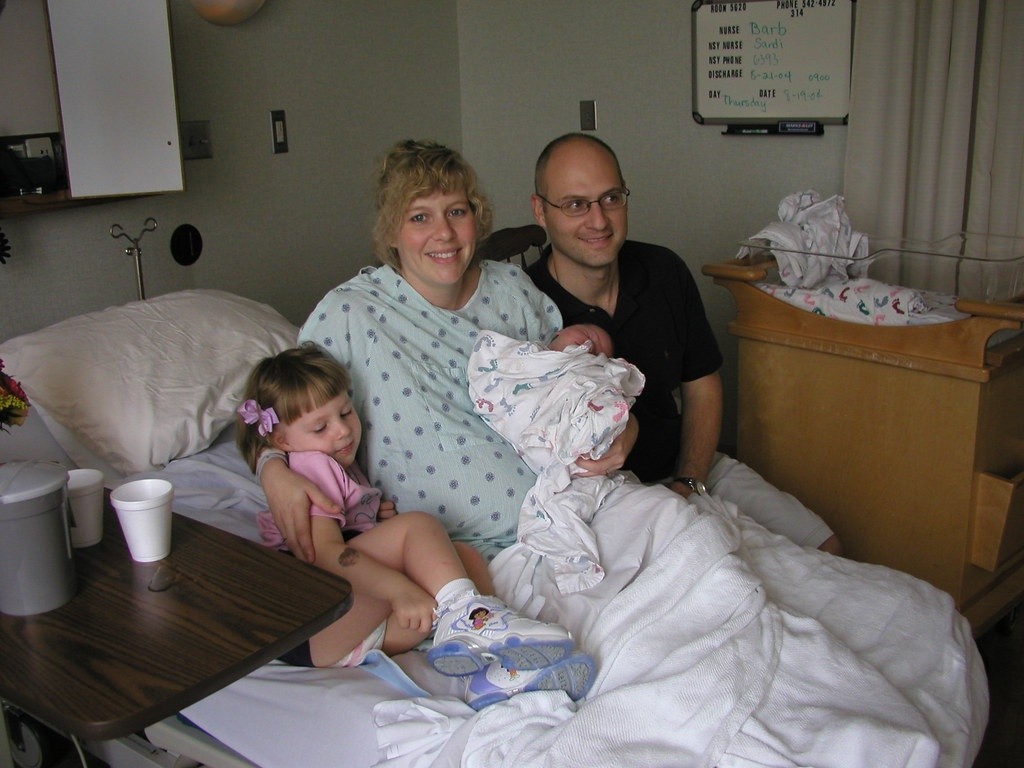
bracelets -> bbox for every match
[255,449,289,479]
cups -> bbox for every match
[65,468,104,548]
[0,462,72,616]
[109,479,174,562]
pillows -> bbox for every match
[0,288,300,478]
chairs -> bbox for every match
[479,224,547,271]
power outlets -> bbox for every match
[270,110,289,154]
[25,137,55,175]
[580,100,597,130]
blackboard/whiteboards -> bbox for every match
[692,0,857,124]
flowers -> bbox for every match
[0,359,31,435]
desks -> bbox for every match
[0,487,354,768]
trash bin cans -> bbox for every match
[0,463,83,616]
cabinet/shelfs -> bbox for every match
[699,255,1024,640]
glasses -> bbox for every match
[536,183,631,216]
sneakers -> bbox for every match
[425,594,574,677]
[463,650,596,712]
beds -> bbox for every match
[0,287,990,768]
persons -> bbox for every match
[520,134,842,556]
[233,342,596,712]
[253,137,939,768]
[468,323,629,536]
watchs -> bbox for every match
[675,475,706,495]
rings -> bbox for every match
[604,470,609,475]
